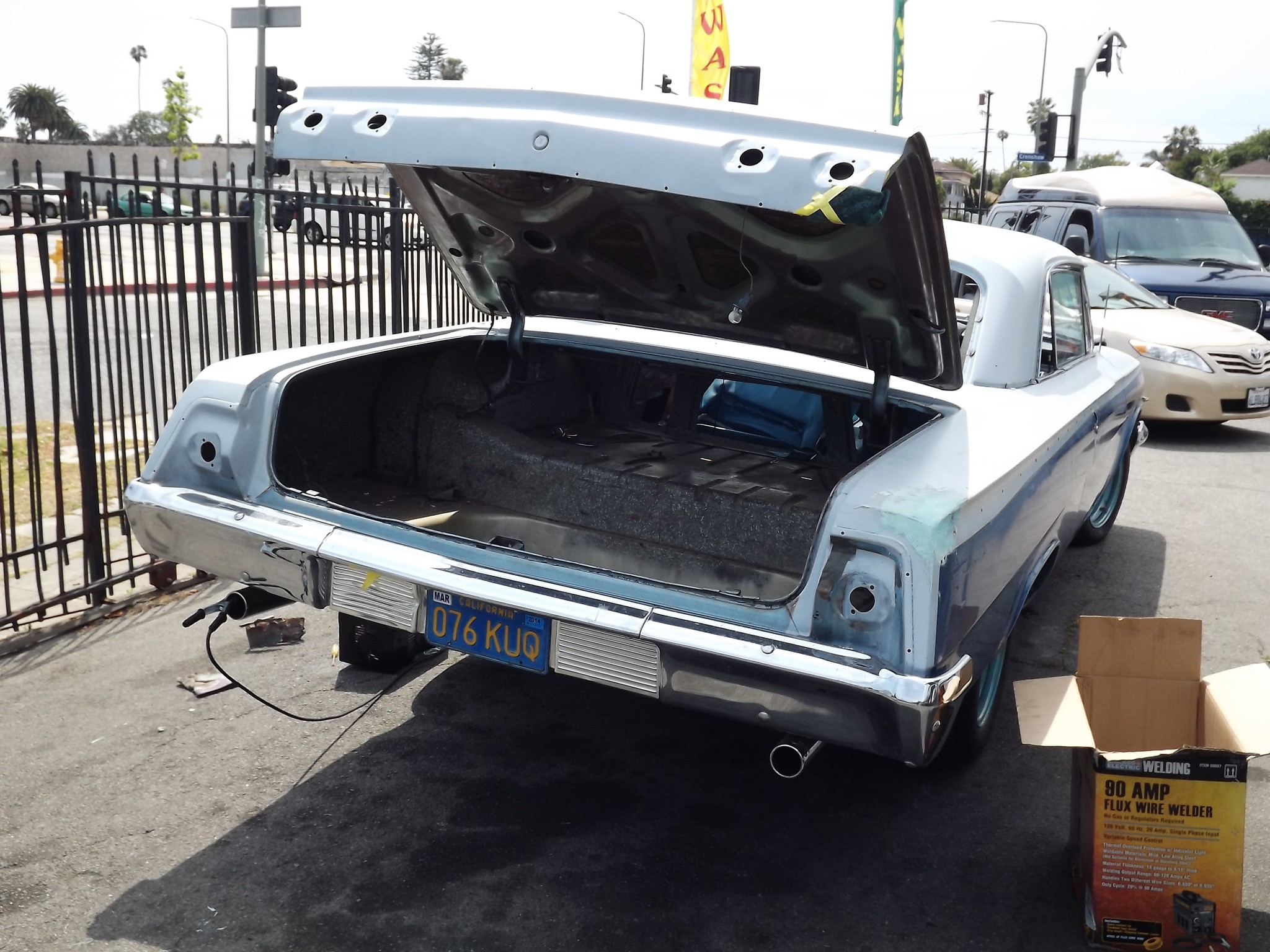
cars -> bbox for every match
[124,88,1163,767]
[951,254,1270,424]
[0,183,93,218]
[240,186,433,250]
[106,190,193,226]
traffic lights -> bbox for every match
[662,73,672,94]
[1039,112,1057,162]
[1096,28,1113,76]
[253,66,298,126]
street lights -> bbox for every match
[187,15,231,215]
[618,11,645,90]
[991,17,1047,176]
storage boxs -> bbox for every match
[1013,616,1270,952]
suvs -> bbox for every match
[983,166,1270,338]
